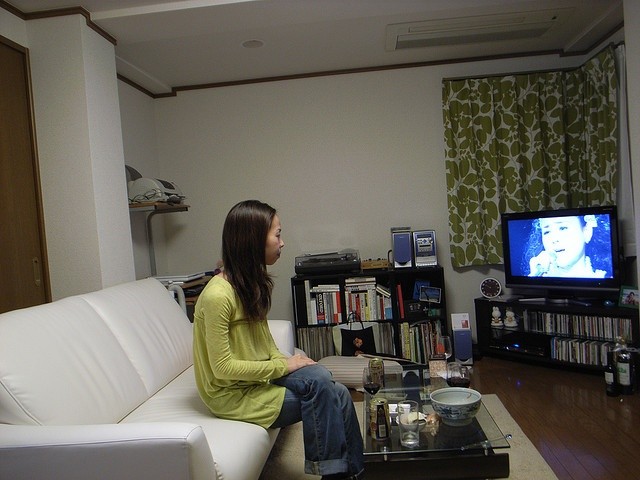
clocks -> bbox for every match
[479,277,502,299]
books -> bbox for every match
[304,281,312,326]
[378,322,396,355]
[345,277,392,321]
[297,326,334,362]
[310,284,342,325]
[551,336,611,366]
[398,321,441,364]
[451,313,470,330]
[524,309,632,341]
[397,285,405,319]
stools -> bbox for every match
[315,354,405,392]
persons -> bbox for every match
[528,215,606,278]
[193,200,365,480]
[625,292,635,306]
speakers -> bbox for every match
[413,229,438,268]
[391,226,412,268]
[453,329,474,365]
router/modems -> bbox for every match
[361,259,389,270]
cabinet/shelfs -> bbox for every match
[290,264,449,370]
[474,293,640,378]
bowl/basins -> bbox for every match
[395,411,429,433]
[429,387,482,427]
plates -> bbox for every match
[387,403,411,418]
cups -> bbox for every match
[397,399,420,450]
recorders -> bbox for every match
[294,249,361,275]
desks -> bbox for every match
[128,202,214,307]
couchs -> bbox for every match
[1,276,309,480]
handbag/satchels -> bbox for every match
[341,316,376,356]
[332,313,379,357]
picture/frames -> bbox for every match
[617,285,640,309]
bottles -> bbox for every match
[368,397,392,441]
[368,358,385,390]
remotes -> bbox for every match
[371,392,408,400]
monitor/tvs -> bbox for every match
[501,205,621,308]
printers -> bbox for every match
[127,177,186,204]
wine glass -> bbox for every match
[362,366,384,398]
[452,365,473,390]
[446,362,462,388]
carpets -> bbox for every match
[257,392,556,480]
